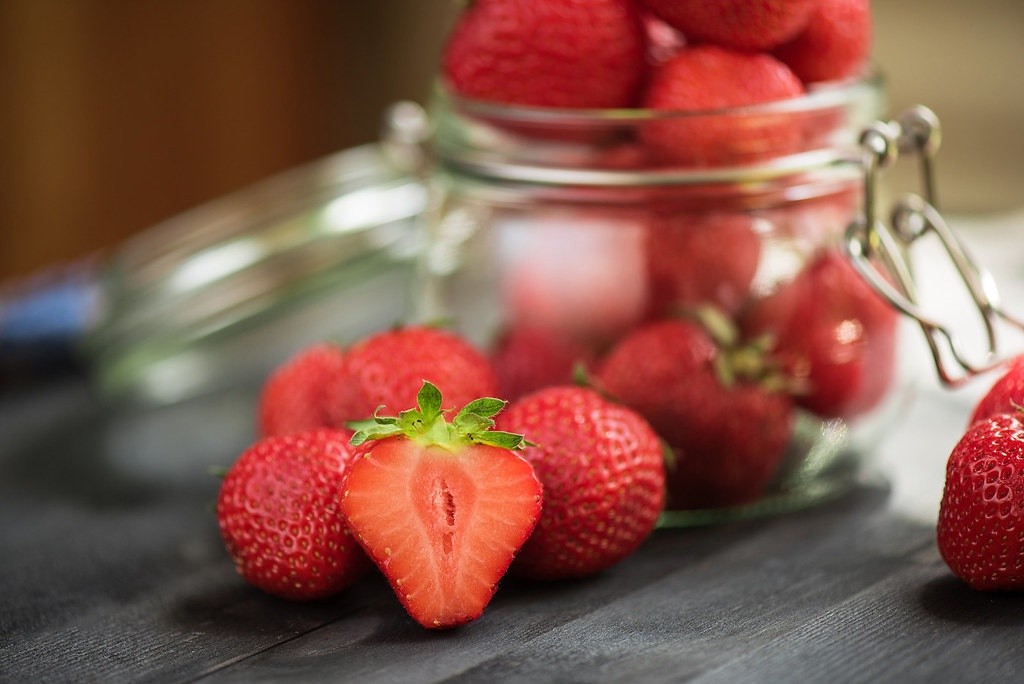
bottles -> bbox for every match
[420,57,902,528]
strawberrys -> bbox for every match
[216,0,902,629]
[934,351,1024,595]
